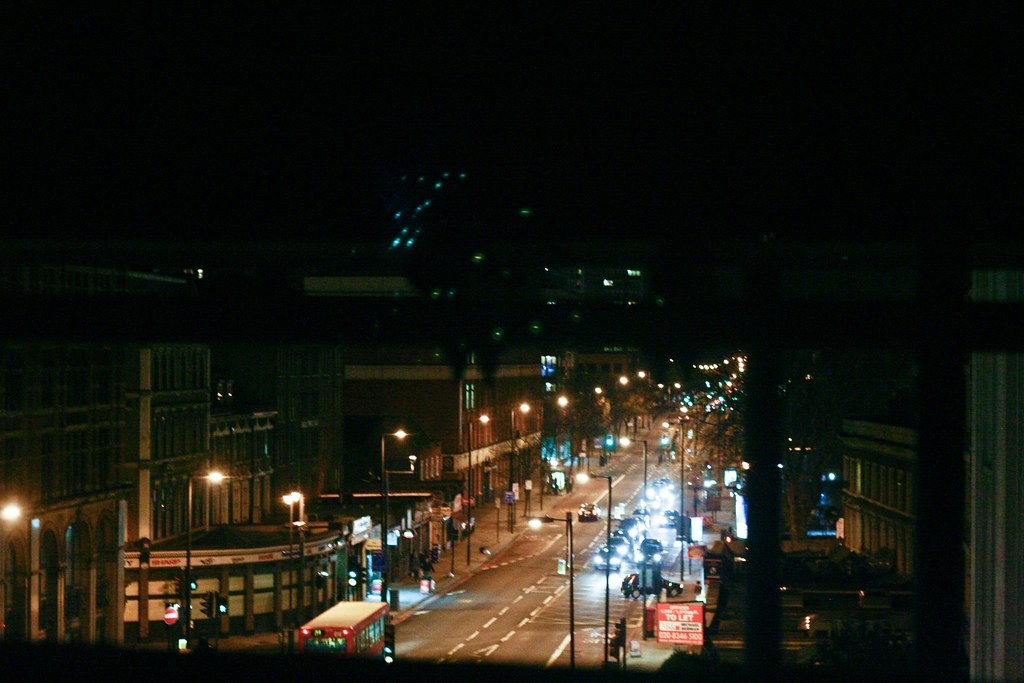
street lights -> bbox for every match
[466,415,489,563]
[662,421,684,583]
[510,404,529,529]
[185,473,228,653]
[528,516,574,665]
[620,435,646,640]
[575,471,612,663]
[280,491,306,649]
[539,396,569,507]
[380,429,409,601]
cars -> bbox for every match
[578,504,602,521]
[445,516,474,538]
[594,508,667,571]
[657,429,713,489]
[551,477,574,494]
[620,573,683,598]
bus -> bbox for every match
[299,599,394,659]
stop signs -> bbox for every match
[163,607,178,624]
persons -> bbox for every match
[411,549,420,582]
[422,556,436,580]
[419,517,475,567]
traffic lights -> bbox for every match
[217,598,227,614]
[382,623,395,664]
[201,593,214,617]
[347,570,359,588]
[175,572,197,595]
